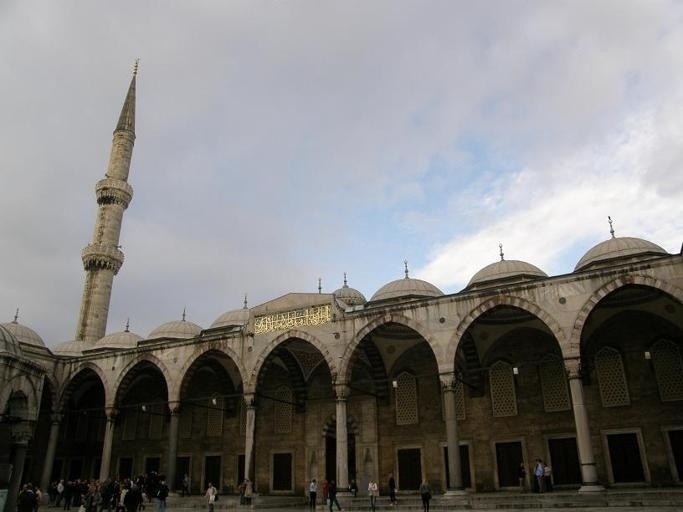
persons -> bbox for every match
[309,478,345,512]
[17,472,169,512]
[202,483,218,512]
[368,479,379,512]
[388,475,397,505]
[519,458,553,494]
[420,479,432,512]
[238,478,253,505]
[349,480,359,497]
[179,473,191,497]
